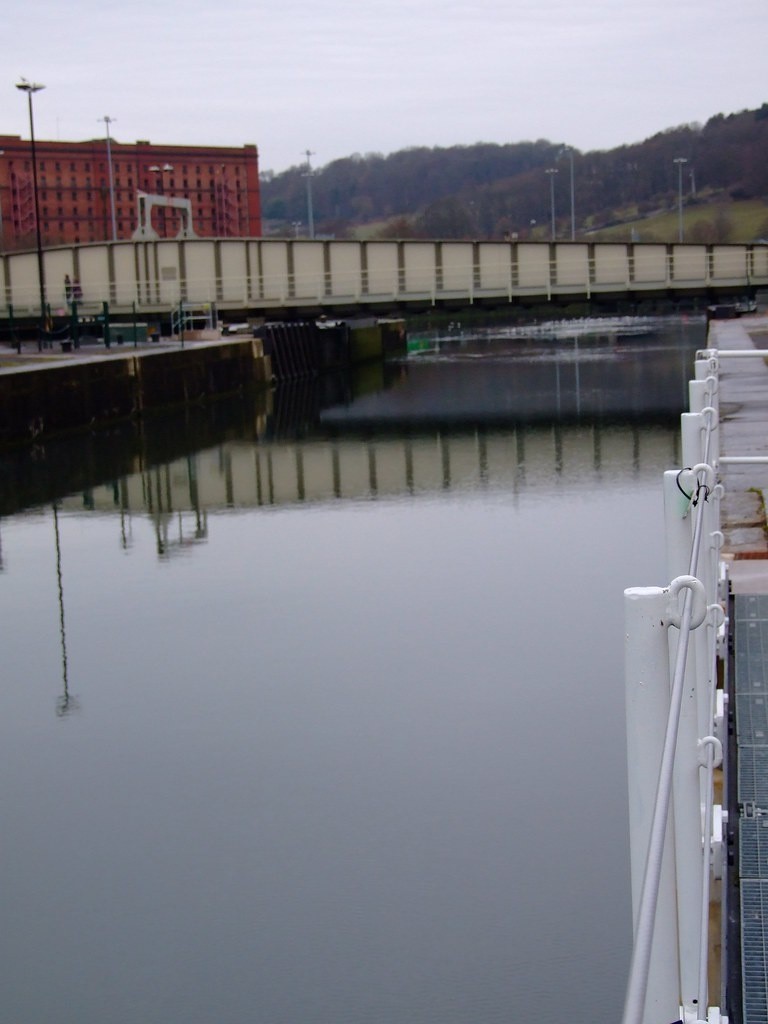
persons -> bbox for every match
[64,275,82,307]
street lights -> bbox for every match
[545,169,559,240]
[292,222,301,240]
[52,501,81,718]
[16,81,50,347]
[673,157,688,242]
[97,116,118,240]
[302,151,317,240]
